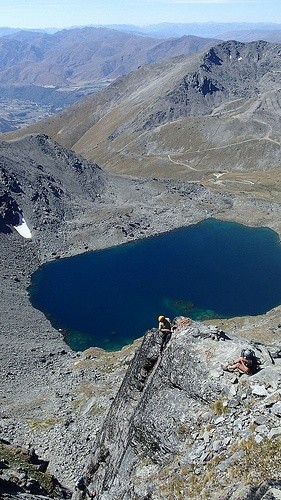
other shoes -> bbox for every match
[165,342,166,346]
[221,364,227,370]
[228,363,232,366]
[160,347,164,351]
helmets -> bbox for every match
[245,351,253,358]
[159,316,165,321]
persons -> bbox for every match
[76,476,94,497]
[221,351,258,375]
[158,315,171,354]
